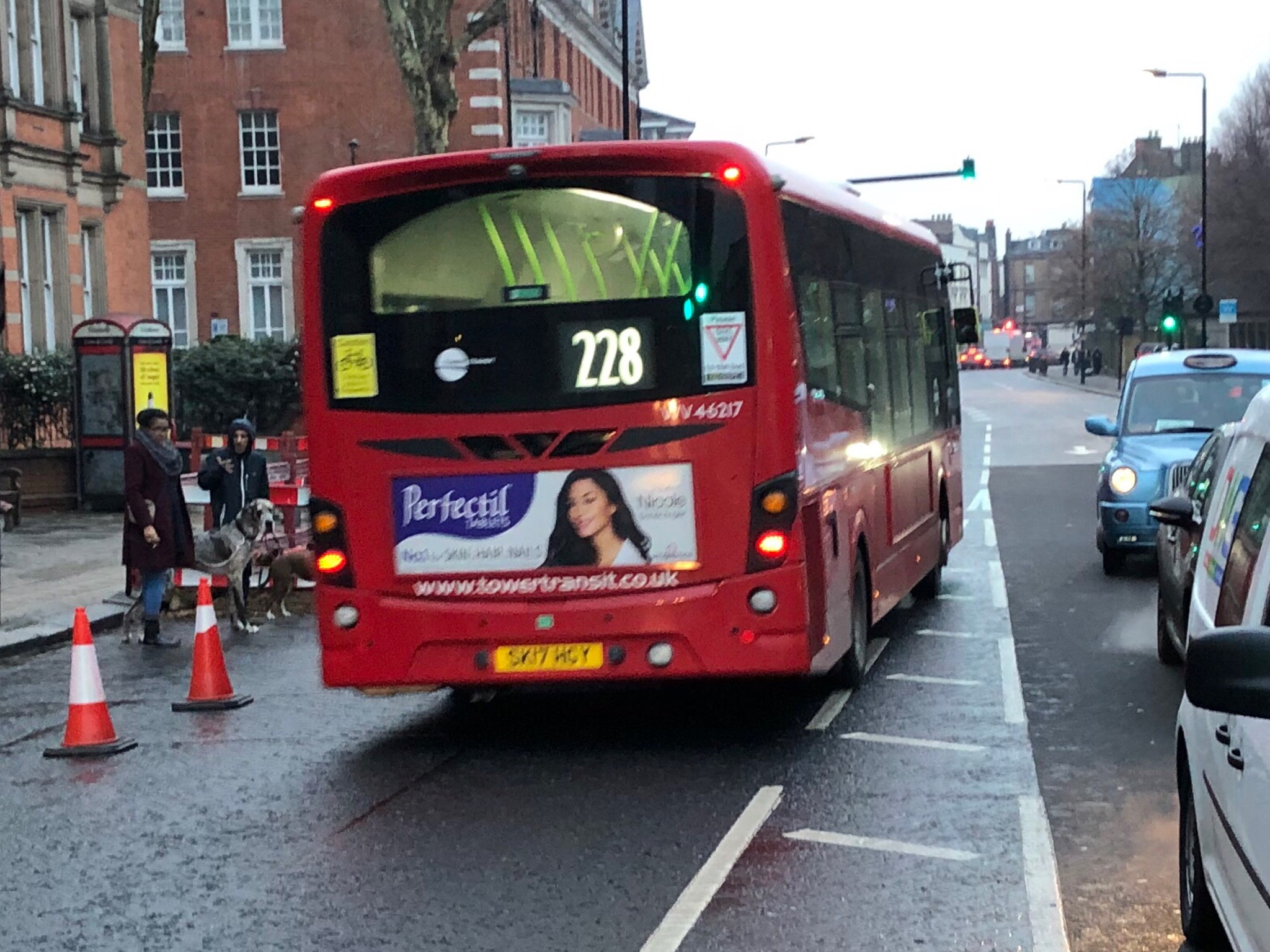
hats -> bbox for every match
[229,417,257,440]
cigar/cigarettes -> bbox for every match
[152,543,157,549]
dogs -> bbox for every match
[122,499,315,641]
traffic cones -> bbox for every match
[41,606,140,758]
[171,578,255,712]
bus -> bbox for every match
[289,138,981,710]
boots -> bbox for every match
[142,616,180,647]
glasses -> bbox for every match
[149,426,170,432]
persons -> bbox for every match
[1059,345,1102,377]
[121,408,198,649]
[531,468,658,568]
[197,419,272,626]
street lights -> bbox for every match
[1143,65,1214,349]
[1057,179,1087,333]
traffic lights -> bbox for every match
[1155,286,1184,336]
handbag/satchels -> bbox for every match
[256,532,283,565]
[128,499,157,522]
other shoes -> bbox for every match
[243,610,268,623]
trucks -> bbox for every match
[983,329,1026,368]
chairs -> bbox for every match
[1153,380,1213,429]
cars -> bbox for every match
[1146,421,1240,665]
[962,345,1062,371]
[1083,345,1270,579]
[1145,379,1270,952]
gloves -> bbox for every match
[216,456,235,474]
